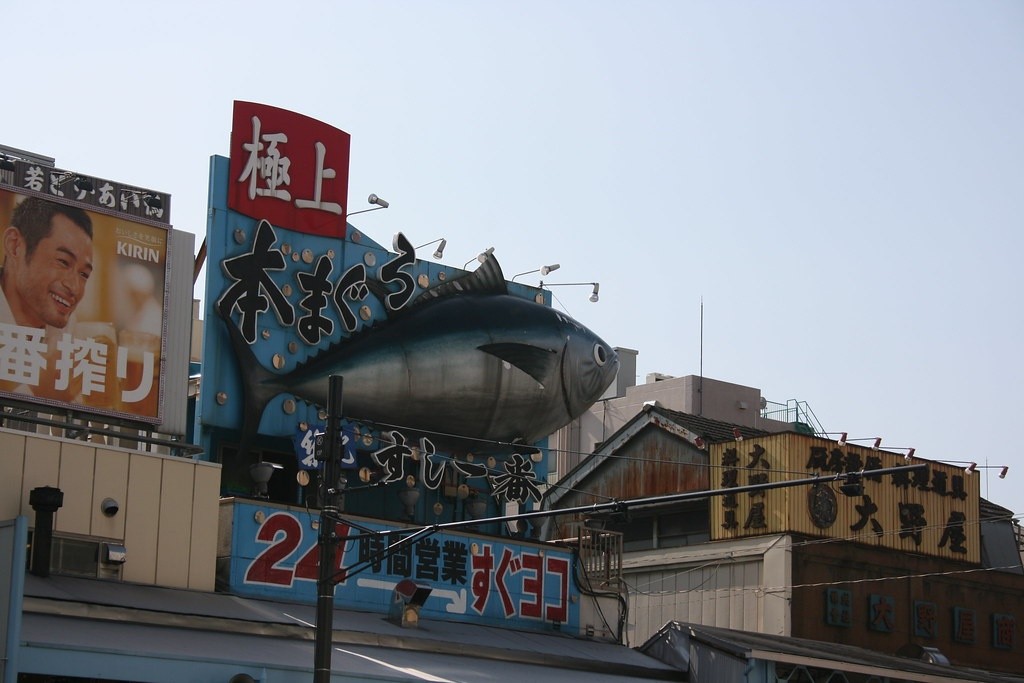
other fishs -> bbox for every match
[217,254,620,466]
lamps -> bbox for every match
[527,509,549,538]
[0,155,17,172]
[930,460,977,474]
[816,432,848,445]
[125,189,163,208]
[467,501,488,527]
[414,238,448,258]
[397,488,421,518]
[540,281,599,302]
[851,437,882,450]
[247,465,275,496]
[980,465,1008,479]
[512,264,561,282]
[463,247,495,269]
[885,447,915,461]
[346,194,388,216]
[52,172,92,191]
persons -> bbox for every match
[0,197,120,441]
